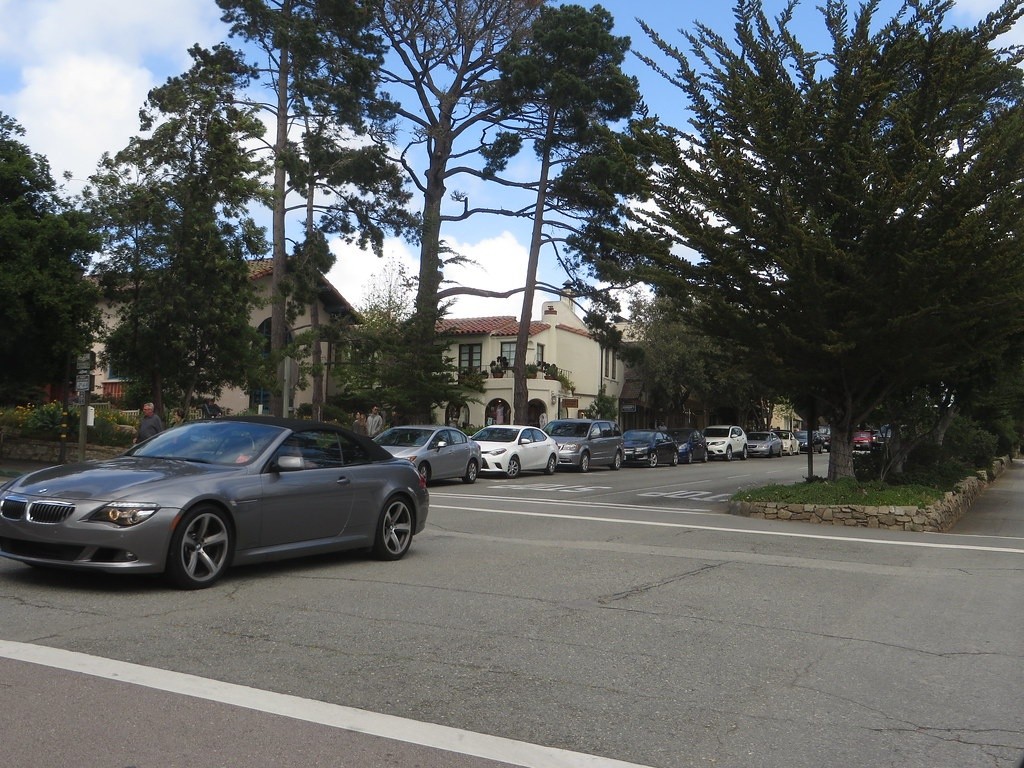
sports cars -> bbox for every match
[0,414,432,589]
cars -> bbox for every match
[467,424,561,478]
[792,431,826,453]
[666,428,709,465]
[773,431,801,456]
[815,426,889,452]
[621,429,680,468]
[542,419,626,472]
[746,431,783,458]
[370,425,483,488]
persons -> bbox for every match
[236,436,322,468]
[132,403,163,444]
[170,409,188,428]
[352,405,383,438]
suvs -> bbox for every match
[701,425,748,461]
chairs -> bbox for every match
[278,456,306,471]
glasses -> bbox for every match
[173,413,178,416]
[374,408,378,410]
[143,409,150,411]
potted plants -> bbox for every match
[489,356,509,378]
[480,370,489,379]
[543,366,557,380]
[525,363,538,379]
